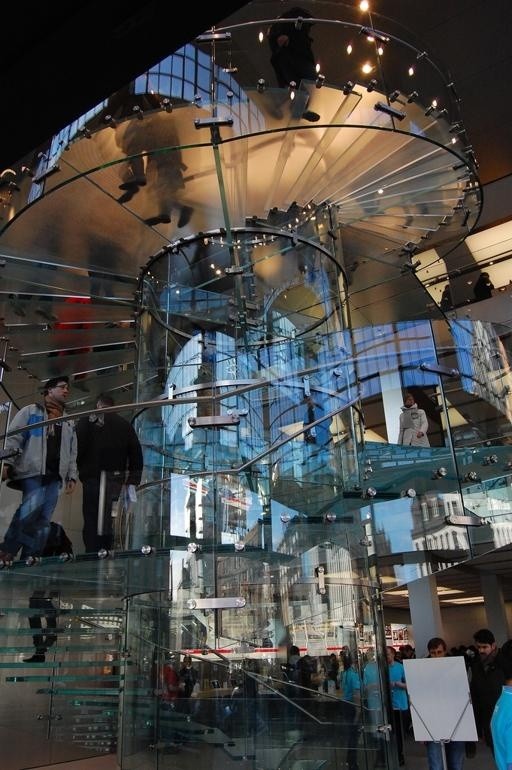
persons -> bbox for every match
[387,626,512,769]
[0,378,79,559]
[396,395,431,446]
[472,271,493,301]
[8,211,127,382]
[69,391,145,552]
[264,7,324,123]
[299,391,334,459]
[145,116,194,229]
[116,121,151,203]
[18,512,72,662]
[156,639,386,769]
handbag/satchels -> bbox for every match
[20,522,73,560]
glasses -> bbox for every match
[56,385,69,391]
